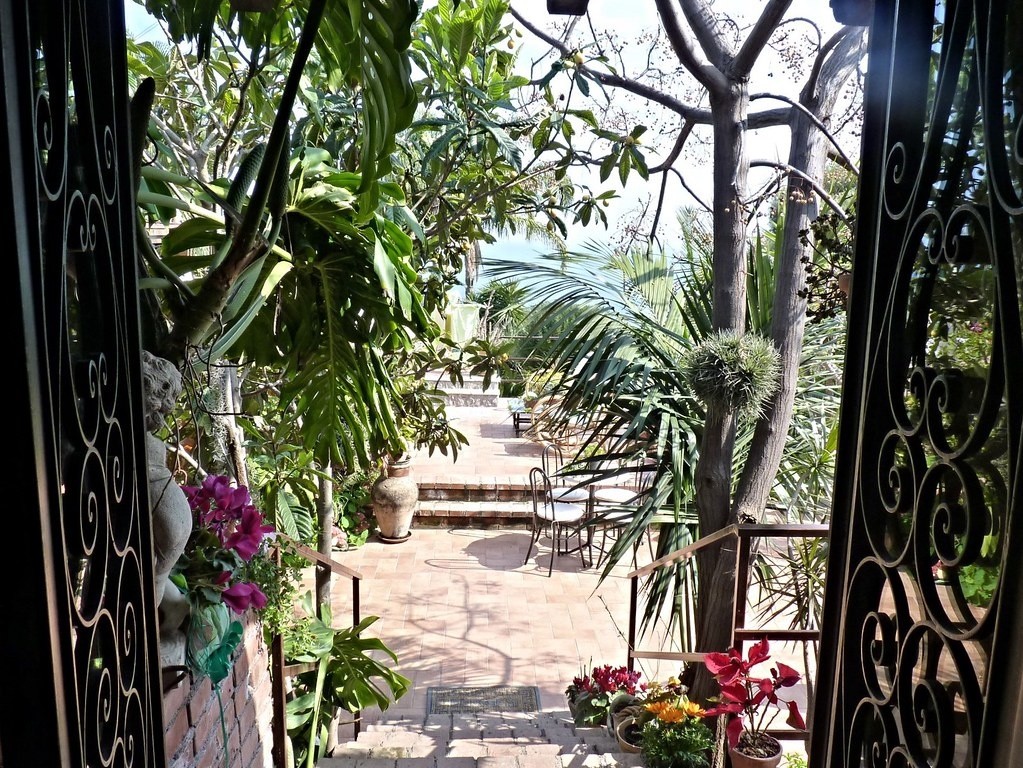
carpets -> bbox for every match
[426,685,542,714]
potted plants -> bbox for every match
[285,588,413,757]
[702,634,806,768]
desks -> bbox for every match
[560,473,634,567]
[508,403,543,438]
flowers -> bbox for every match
[564,656,648,729]
[630,676,688,747]
[169,473,276,614]
[638,694,717,768]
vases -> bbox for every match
[616,718,640,753]
[568,697,577,720]
[371,462,419,544]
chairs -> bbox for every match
[524,443,655,577]
